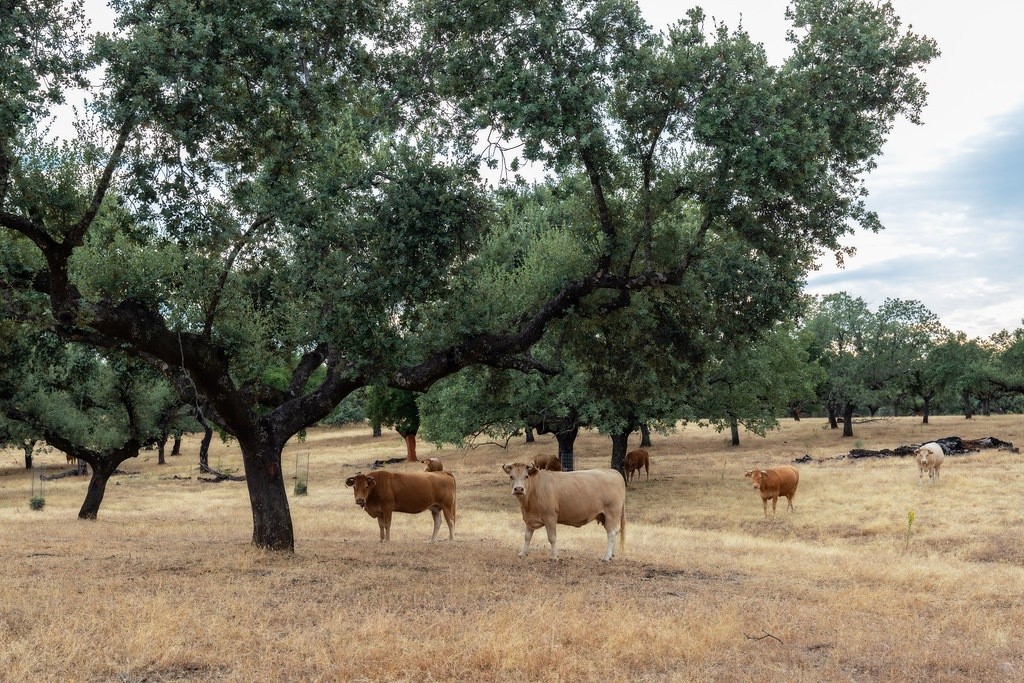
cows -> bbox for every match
[532,454,560,470]
[745,465,799,516]
[501,462,626,564]
[420,457,443,471]
[624,449,650,481]
[914,442,945,490]
[345,470,457,542]
